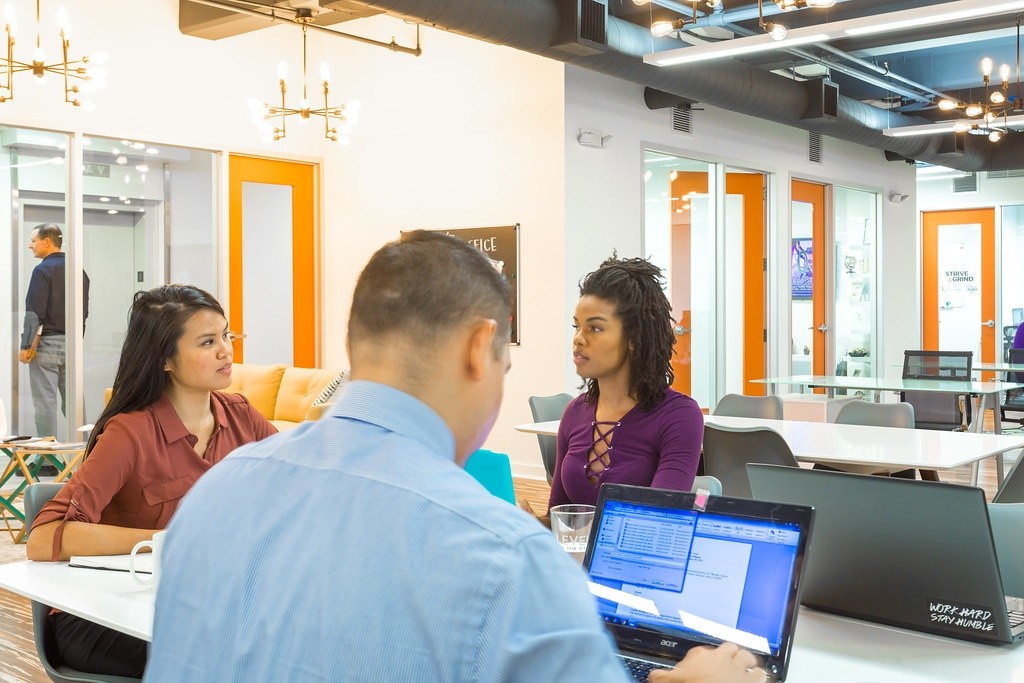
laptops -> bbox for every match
[582,482,817,683]
[746,464,1024,644]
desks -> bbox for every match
[889,361,1024,377]
[0,560,1024,683]
[749,374,1024,493]
[513,414,1024,471]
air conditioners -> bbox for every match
[11,148,166,215]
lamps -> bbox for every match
[260,9,359,146]
[0,0,104,110]
[578,126,616,149]
[632,0,838,42]
[937,13,1024,144]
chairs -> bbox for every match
[1000,324,1024,426]
[527,393,575,487]
[712,392,784,421]
[900,350,974,431]
[985,502,1024,600]
[23,481,143,683]
[702,420,801,500]
[817,399,916,477]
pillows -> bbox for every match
[274,366,343,423]
[224,363,291,422]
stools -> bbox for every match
[0,435,87,544]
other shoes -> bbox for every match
[15,463,60,478]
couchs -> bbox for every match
[104,388,335,432]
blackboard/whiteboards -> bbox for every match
[400,223,520,346]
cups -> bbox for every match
[550,504,596,568]
[129,531,166,595]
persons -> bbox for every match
[25,283,280,679]
[1013,322,1023,391]
[15,223,90,477]
[139,232,771,683]
[536,246,705,544]
